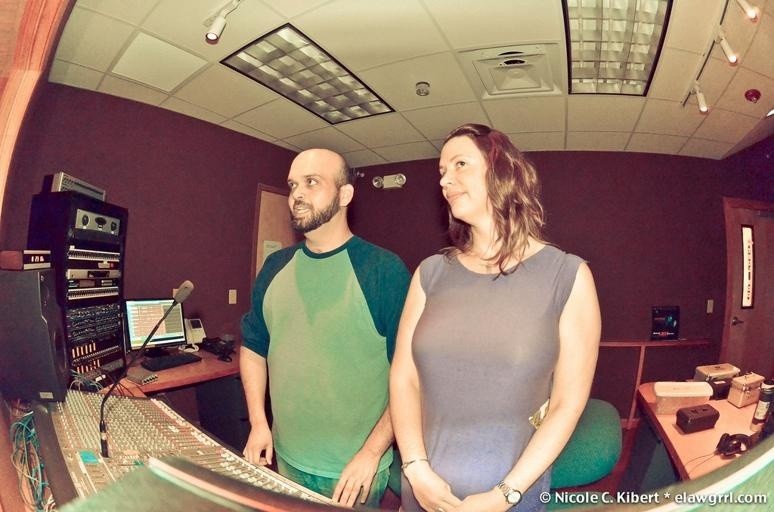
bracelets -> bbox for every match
[401,459,432,470]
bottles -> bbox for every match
[750,380,774,432]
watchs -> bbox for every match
[497,480,522,506]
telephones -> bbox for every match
[184,318,206,344]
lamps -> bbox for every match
[692,84,708,113]
[717,35,737,64]
[736,0,757,19]
[205,13,227,43]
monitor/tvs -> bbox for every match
[124,296,187,357]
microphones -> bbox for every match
[100,279,195,457]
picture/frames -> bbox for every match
[250,184,305,287]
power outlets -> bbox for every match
[707,301,714,313]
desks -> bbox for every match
[637,382,764,483]
[98,346,239,426]
[589,339,711,430]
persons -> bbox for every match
[237,148,414,508]
[387,123,603,512]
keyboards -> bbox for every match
[141,352,202,372]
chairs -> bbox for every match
[550,399,625,488]
[388,448,401,496]
